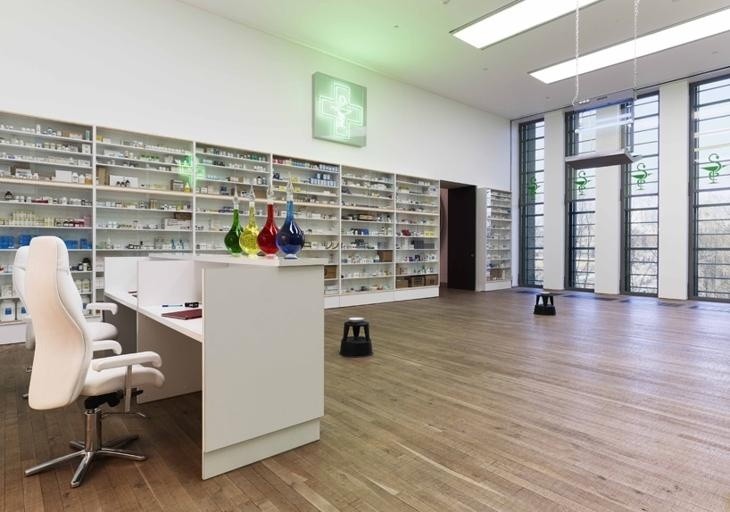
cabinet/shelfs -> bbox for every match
[478,187,512,292]
[272,153,341,310]
[0,108,95,346]
[94,123,194,322]
[395,173,441,302]
[194,140,272,256]
[340,164,395,308]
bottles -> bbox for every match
[225,178,305,259]
[0,120,191,321]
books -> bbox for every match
[161,308,202,320]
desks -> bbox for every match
[101,251,329,480]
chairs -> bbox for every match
[12,236,166,488]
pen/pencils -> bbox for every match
[162,305,184,307]
